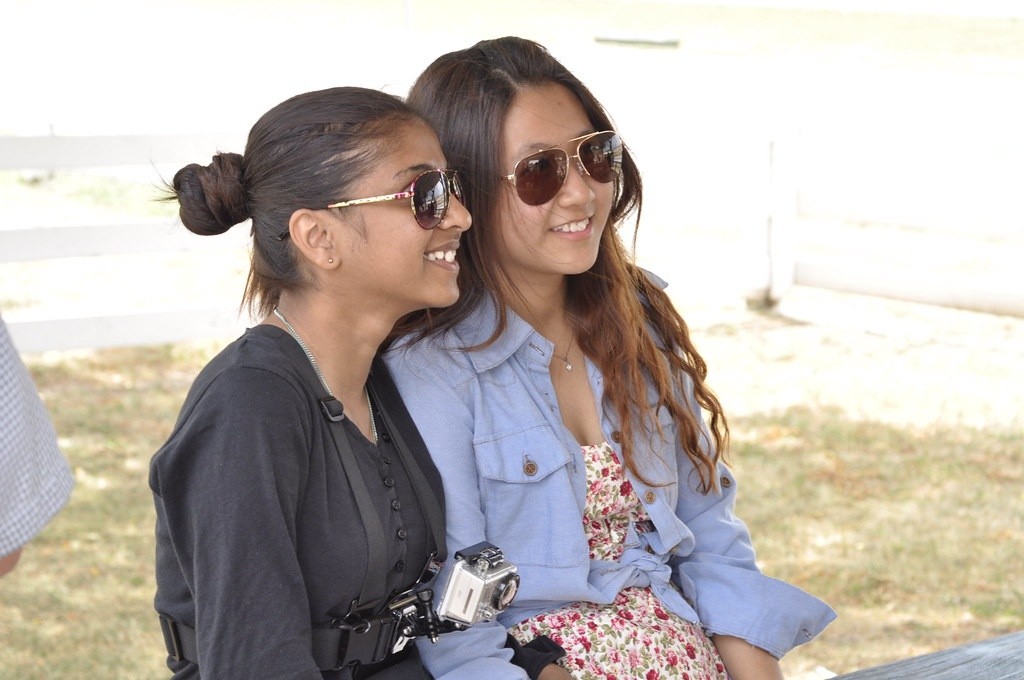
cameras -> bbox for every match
[437,540,521,626]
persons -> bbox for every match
[381,36,787,680]
[147,86,474,680]
[0,308,55,583]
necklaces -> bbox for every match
[551,326,576,372]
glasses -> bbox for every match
[275,169,466,242]
[495,130,624,207]
